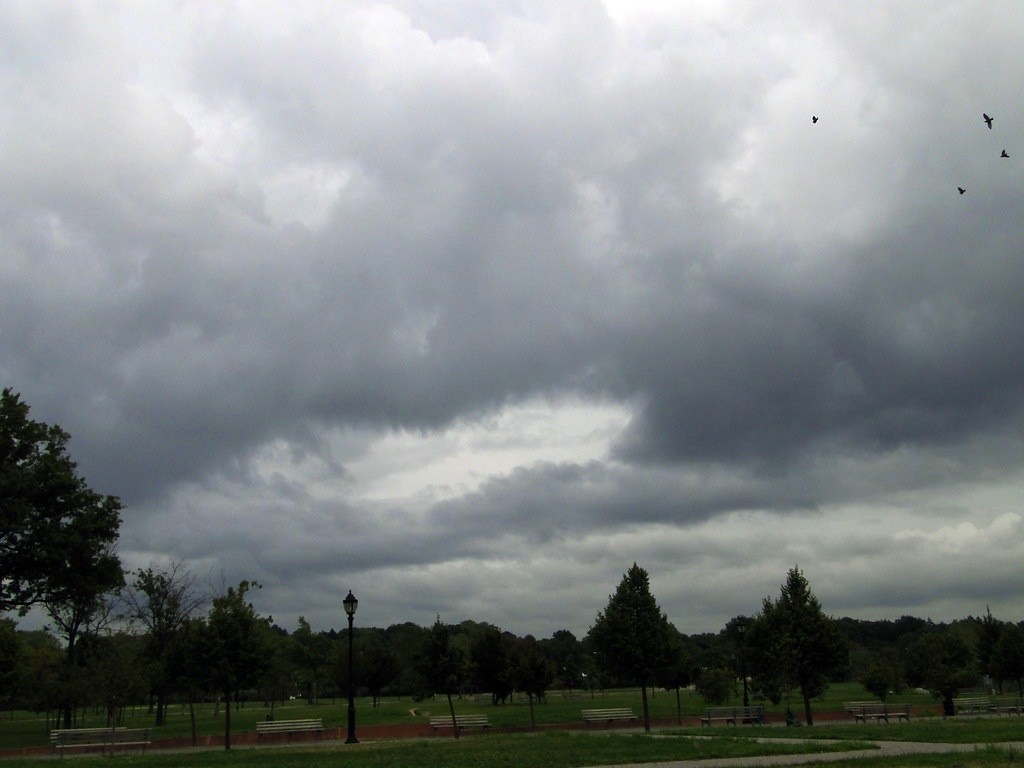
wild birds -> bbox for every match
[811,115,819,124]
[999,148,1010,158]
[958,186,966,195]
[982,113,994,130]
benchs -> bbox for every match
[56,728,154,759]
[255,718,325,744]
[855,702,911,725]
[50,727,128,755]
[952,697,994,715]
[843,700,887,720]
[700,706,761,728]
[581,707,639,731]
[991,698,1024,716]
[429,714,493,738]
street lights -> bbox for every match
[736,618,752,724]
[340,588,361,745]
